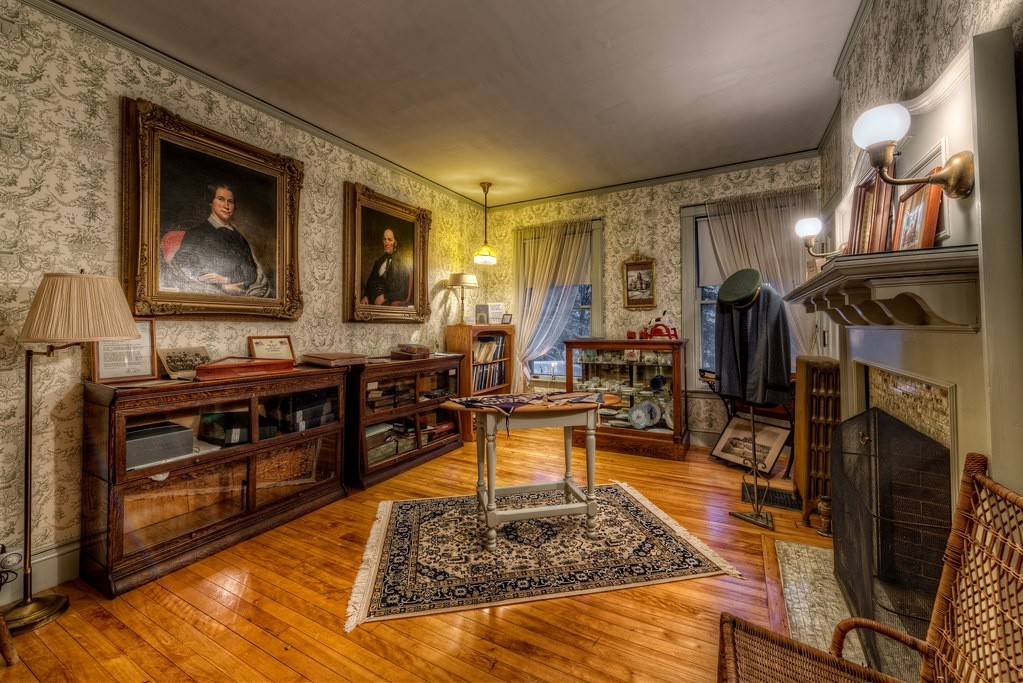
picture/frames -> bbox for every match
[622,248,657,311]
[846,135,950,252]
[122,96,306,320]
[342,180,433,325]
[709,415,791,475]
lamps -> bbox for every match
[2,270,141,639]
[851,103,974,200]
[474,182,497,265]
[793,217,849,257]
[445,272,479,325]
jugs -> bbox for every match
[641,349,673,366]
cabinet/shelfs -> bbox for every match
[563,338,691,462]
[446,324,516,442]
[80,365,350,600]
[347,353,467,490]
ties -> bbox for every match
[739,312,747,395]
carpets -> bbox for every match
[342,480,743,634]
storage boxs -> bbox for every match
[125,421,194,470]
[367,434,427,464]
[475,302,505,325]
[390,343,430,360]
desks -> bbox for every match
[440,392,621,552]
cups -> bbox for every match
[577,376,634,396]
[578,348,621,363]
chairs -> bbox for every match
[717,453,1023,683]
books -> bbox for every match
[473,336,505,392]
[390,342,430,359]
[197,399,335,446]
[301,352,368,367]
[364,368,456,464]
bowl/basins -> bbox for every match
[639,390,655,396]
[586,388,609,394]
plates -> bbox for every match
[598,400,676,431]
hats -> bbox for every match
[718,269,761,311]
